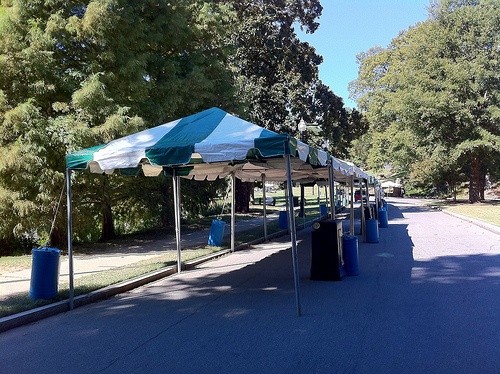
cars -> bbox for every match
[354,189,370,202]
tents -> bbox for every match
[65,106,333,316]
[178,107,386,316]
